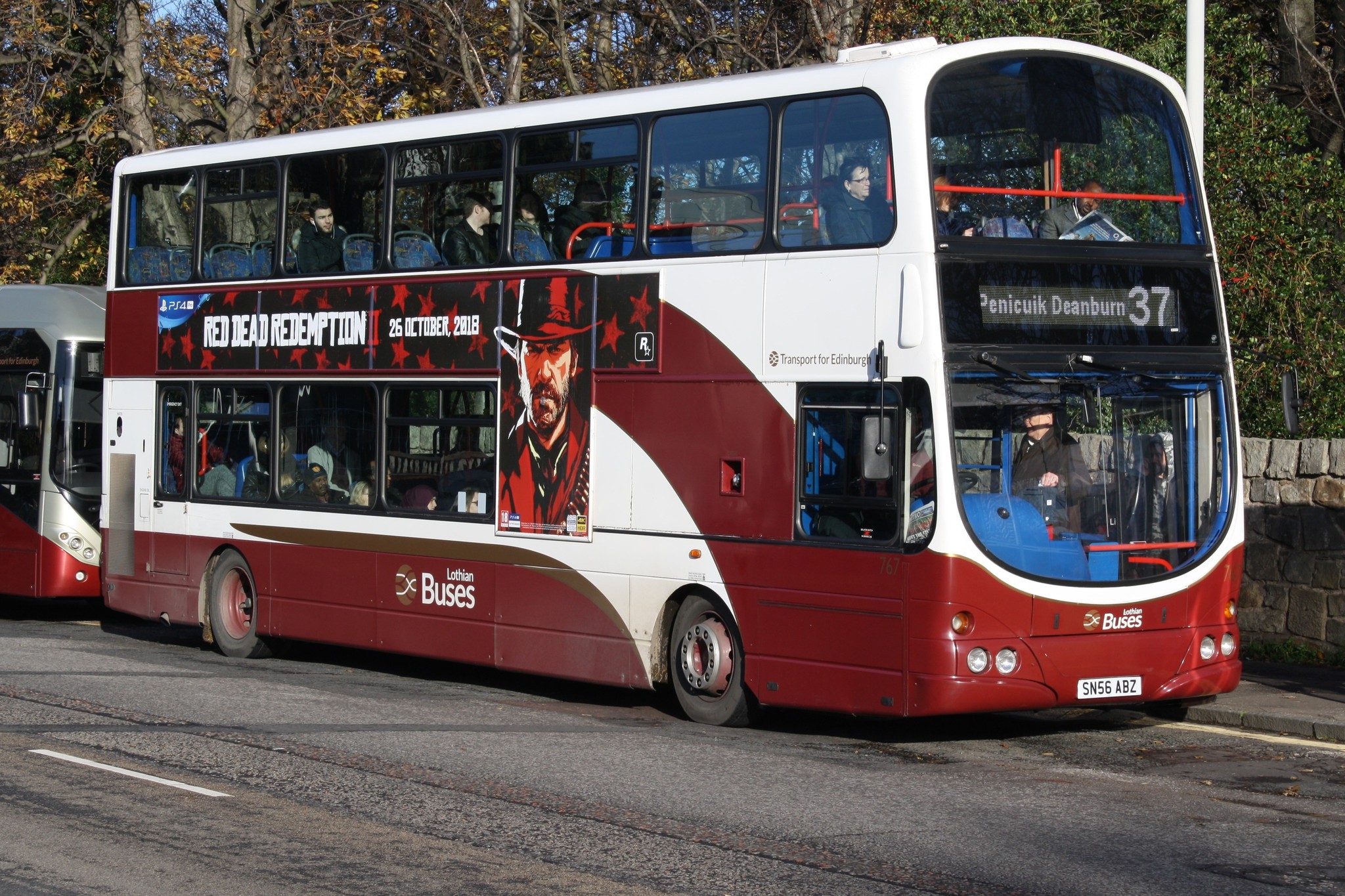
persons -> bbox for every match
[812,395,1177,579]
[290,182,608,280]
[817,159,1108,248]
[169,419,481,513]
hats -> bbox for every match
[305,462,327,486]
[1014,404,1061,417]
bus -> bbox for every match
[97,35,1302,724]
[2,282,108,599]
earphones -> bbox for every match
[313,221,315,226]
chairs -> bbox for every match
[128,182,821,288]
[163,409,489,513]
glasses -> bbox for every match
[850,176,870,184]
[951,195,964,202]
[1019,412,1046,422]
[472,500,478,506]
